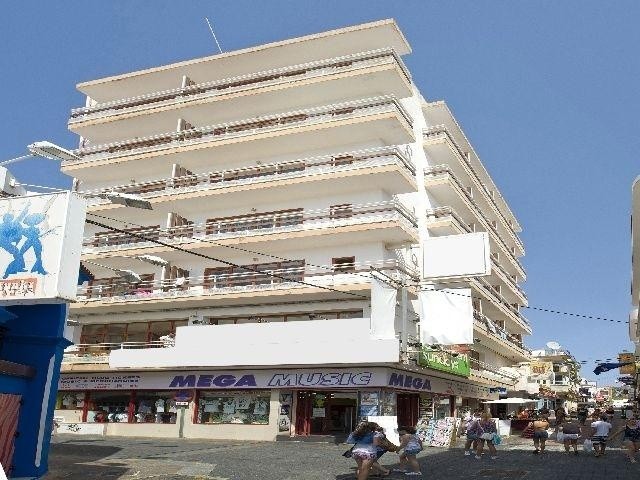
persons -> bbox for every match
[459,400,640,463]
[346,420,425,480]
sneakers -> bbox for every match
[465,451,471,456]
[407,472,422,476]
[475,455,481,460]
[393,469,406,472]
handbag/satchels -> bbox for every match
[482,433,493,440]
[342,450,351,458]
[493,433,500,445]
